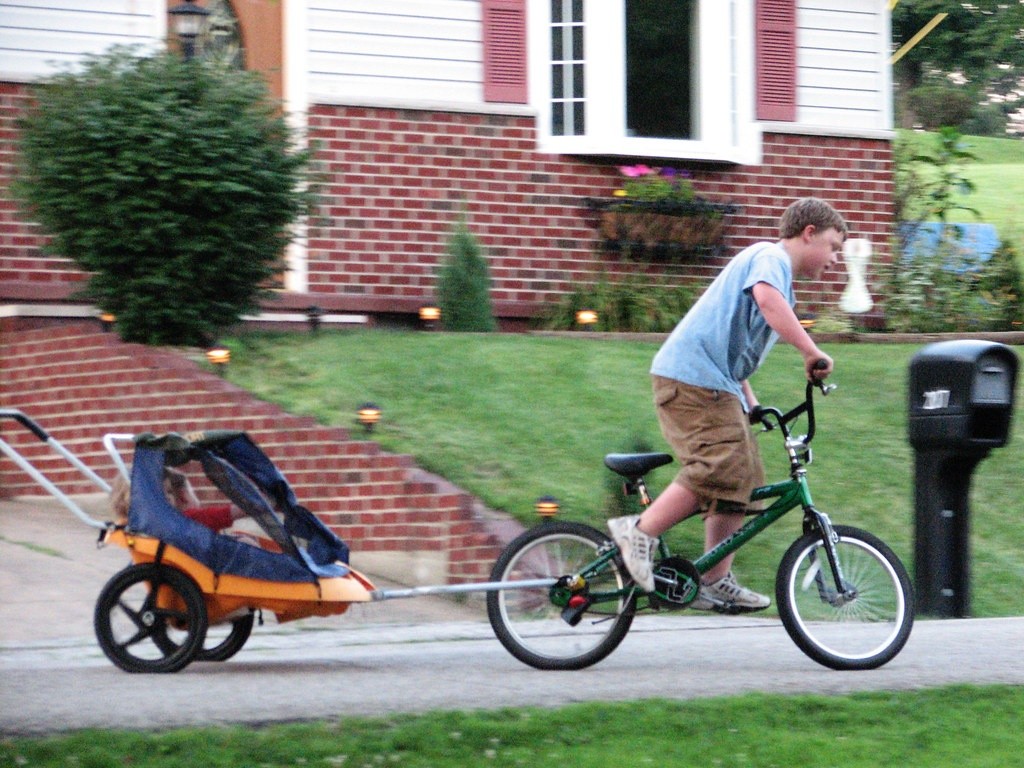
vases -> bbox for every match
[589,198,736,258]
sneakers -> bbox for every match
[690,571,771,612]
[607,513,659,592]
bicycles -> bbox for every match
[486,359,917,672]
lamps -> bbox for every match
[798,313,815,330]
[306,305,321,318]
[359,404,380,424]
[101,310,116,321]
[577,310,598,324]
[207,346,230,364]
[419,303,440,320]
[536,494,558,516]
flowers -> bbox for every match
[612,165,693,201]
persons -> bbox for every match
[607,196,850,615]
[110,471,259,545]
